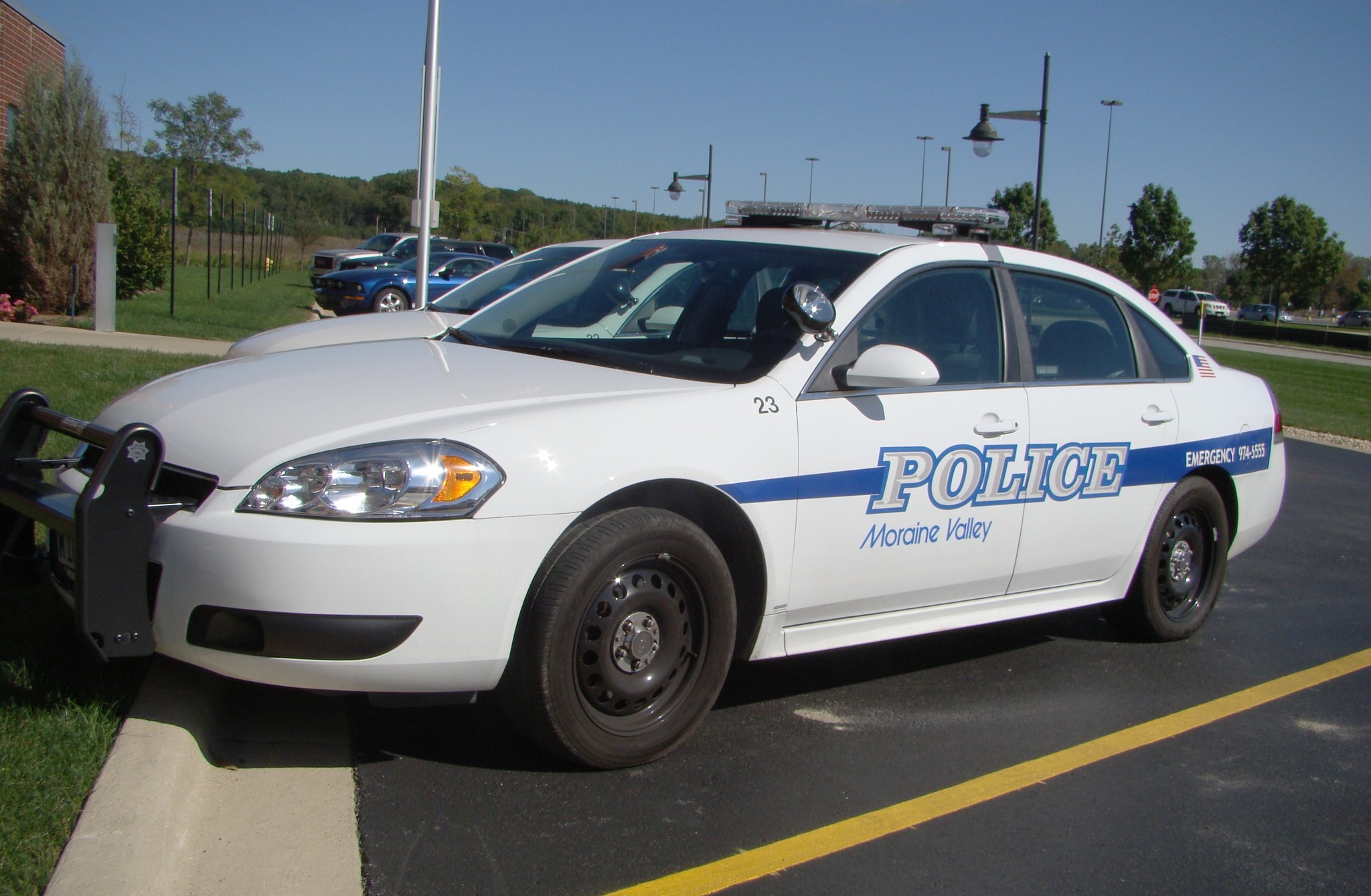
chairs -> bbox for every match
[1036,317,1118,378]
[920,282,981,376]
[750,283,796,351]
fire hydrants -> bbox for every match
[264,257,274,272]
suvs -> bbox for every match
[338,237,520,277]
[310,231,448,287]
[1159,288,1230,321]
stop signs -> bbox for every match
[1148,289,1159,304]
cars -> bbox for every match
[0,198,1288,769]
[1236,303,1293,323]
[225,238,886,359]
[312,250,534,318]
[1337,311,1371,329]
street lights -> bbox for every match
[611,198,620,232]
[632,200,638,237]
[521,217,526,245]
[602,205,608,239]
[917,135,934,237]
[805,157,820,203]
[571,210,577,241]
[699,189,705,228]
[760,172,767,201]
[1098,99,1123,269]
[664,143,714,228]
[941,147,952,207]
[540,213,545,232]
[650,186,660,233]
[961,52,1053,326]
[509,221,513,244]
